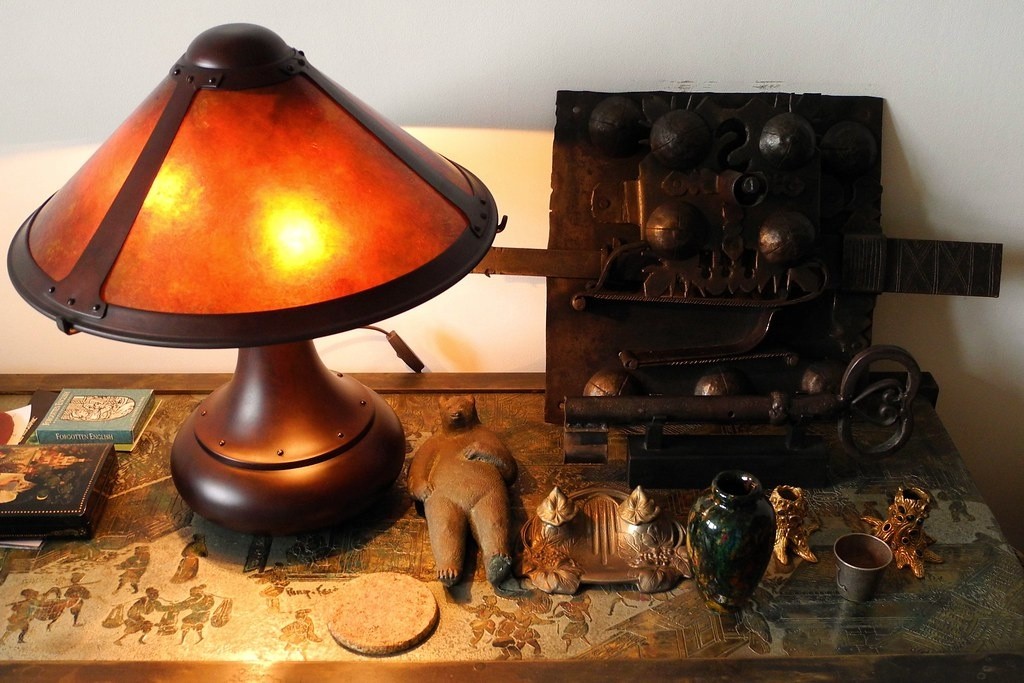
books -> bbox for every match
[18,387,162,452]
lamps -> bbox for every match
[7,23,508,536]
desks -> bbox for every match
[0,372,1024,683]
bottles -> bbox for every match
[685,469,777,617]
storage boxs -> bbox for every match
[36,387,155,445]
[0,443,120,541]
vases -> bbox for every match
[685,471,776,615]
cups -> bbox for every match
[834,532,893,603]
